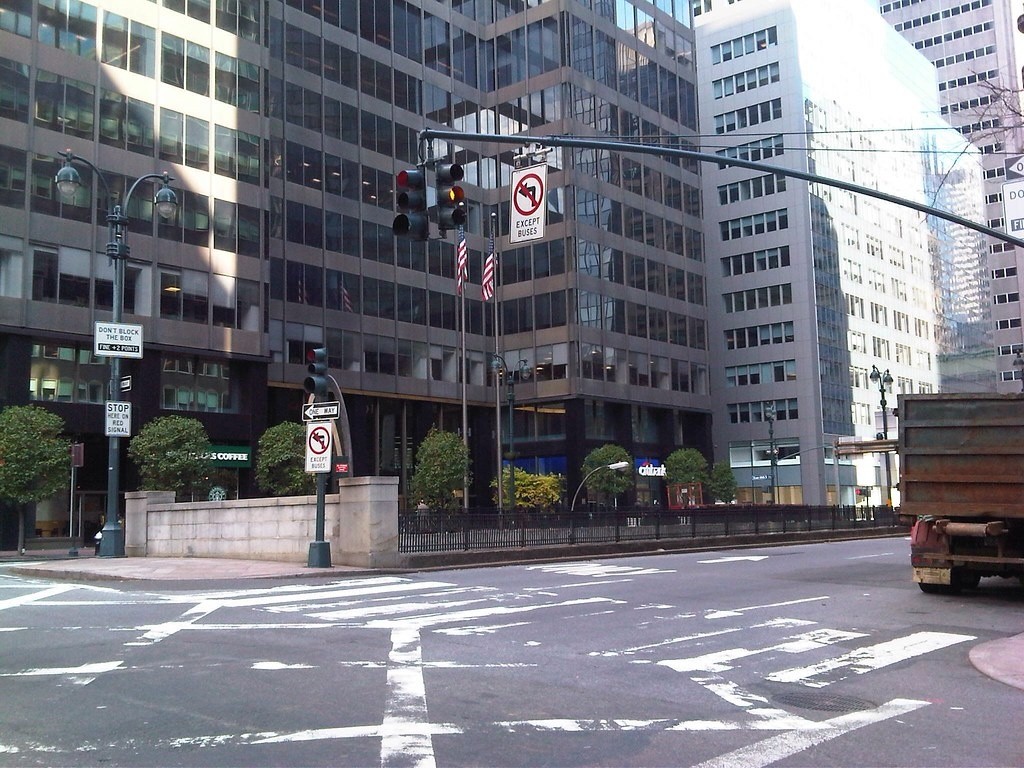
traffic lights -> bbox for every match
[856,489,867,496]
[304,345,329,400]
[393,165,430,243]
[435,161,465,230]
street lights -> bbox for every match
[57,146,181,557]
[566,461,629,546]
[763,402,779,507]
[491,353,533,529]
[1012,349,1024,391]
[870,364,894,513]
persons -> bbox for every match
[418,499,429,529]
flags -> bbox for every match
[456,225,469,295]
[482,228,498,300]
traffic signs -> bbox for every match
[302,400,341,420]
[109,375,132,393]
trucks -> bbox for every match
[891,389,1024,597]
